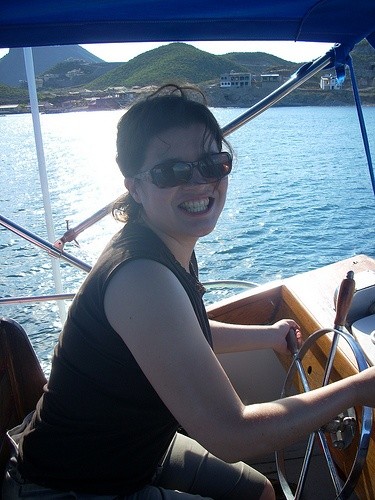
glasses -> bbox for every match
[132,152,233,189]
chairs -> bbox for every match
[0,317,48,487]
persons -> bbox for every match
[17,84,375,500]
